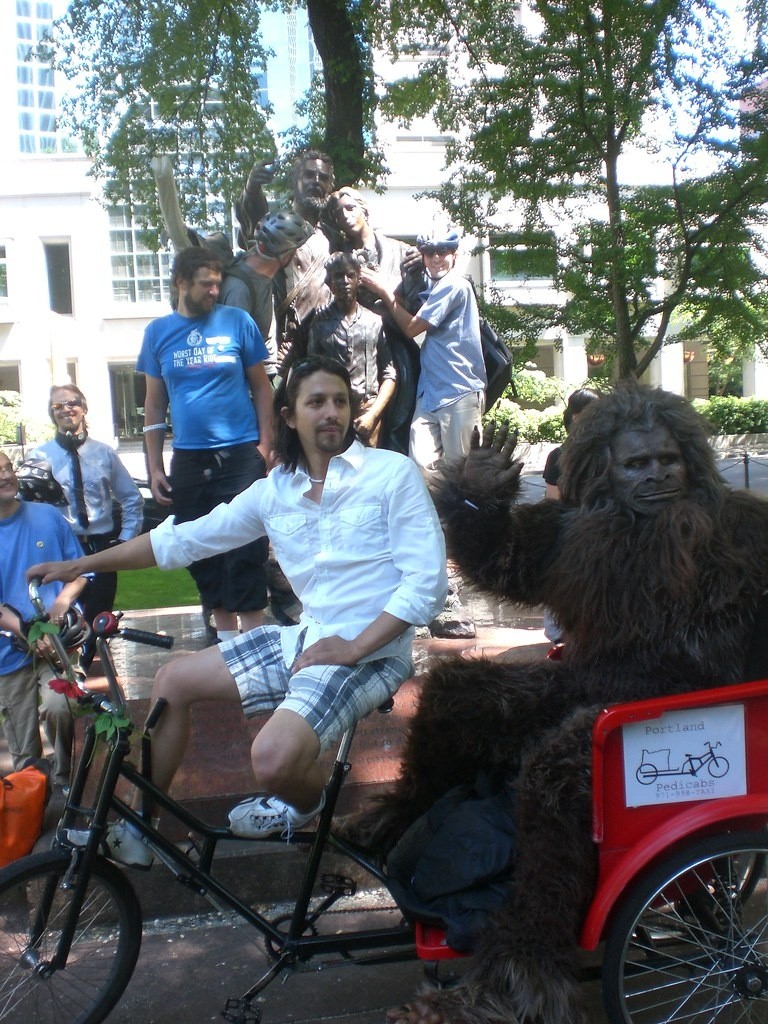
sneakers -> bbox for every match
[61,784,70,797]
[224,788,325,845]
[56,818,154,872]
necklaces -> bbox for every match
[306,464,326,483]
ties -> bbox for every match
[71,449,89,530]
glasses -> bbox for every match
[0,467,19,476]
[49,401,79,409]
[425,245,450,254]
[283,357,347,407]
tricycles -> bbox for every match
[0,573,768,1024]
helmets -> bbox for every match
[416,225,458,251]
[254,209,315,254]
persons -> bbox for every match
[26,384,145,680]
[217,209,314,404]
[360,226,488,528]
[331,382,768,1024]
[233,150,433,457]
[25,355,449,872]
[0,451,95,799]
[542,388,604,645]
[134,247,273,646]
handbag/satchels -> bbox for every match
[476,318,512,417]
